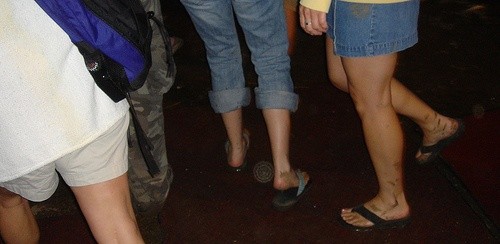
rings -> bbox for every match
[304,20,311,27]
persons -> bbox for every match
[178,0,311,211]
[127,0,179,244]
[299,0,459,230]
[0,0,152,244]
[283,0,297,54]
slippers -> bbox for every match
[272,169,312,212]
[224,128,252,173]
[336,202,413,233]
[415,119,465,165]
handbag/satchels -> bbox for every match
[32,1,154,103]
